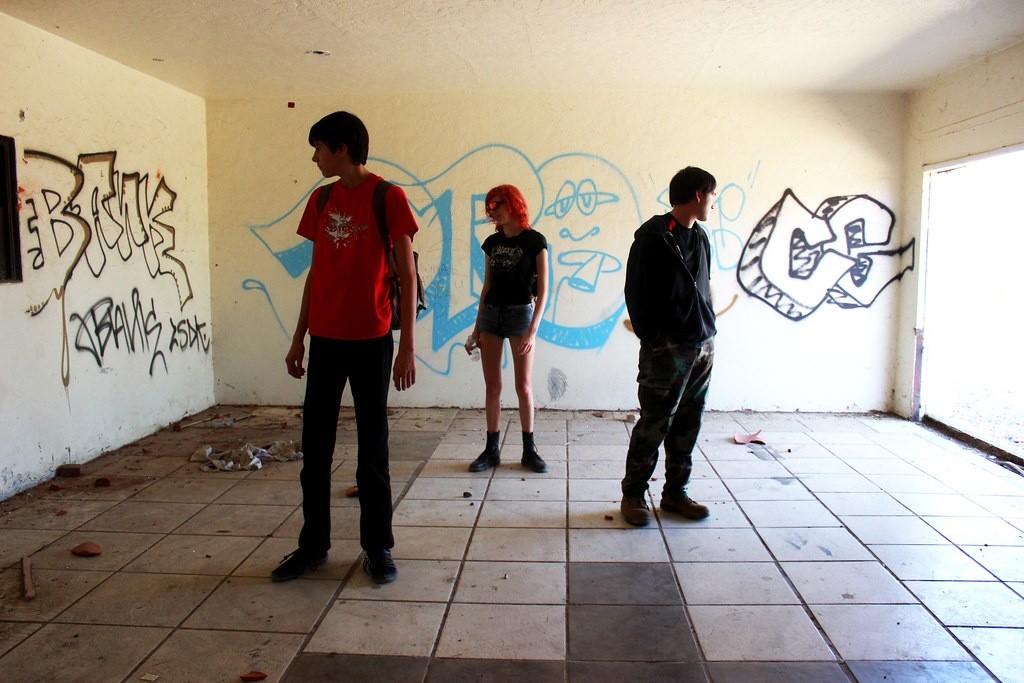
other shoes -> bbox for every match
[660,497,710,521]
[621,497,652,528]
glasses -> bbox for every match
[487,200,504,211]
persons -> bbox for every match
[463,186,550,474]
[271,112,419,583]
[619,166,718,527]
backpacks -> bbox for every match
[320,181,427,330]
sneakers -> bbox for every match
[363,549,397,585]
[272,549,328,583]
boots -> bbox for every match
[468,429,501,473]
[521,431,550,473]
